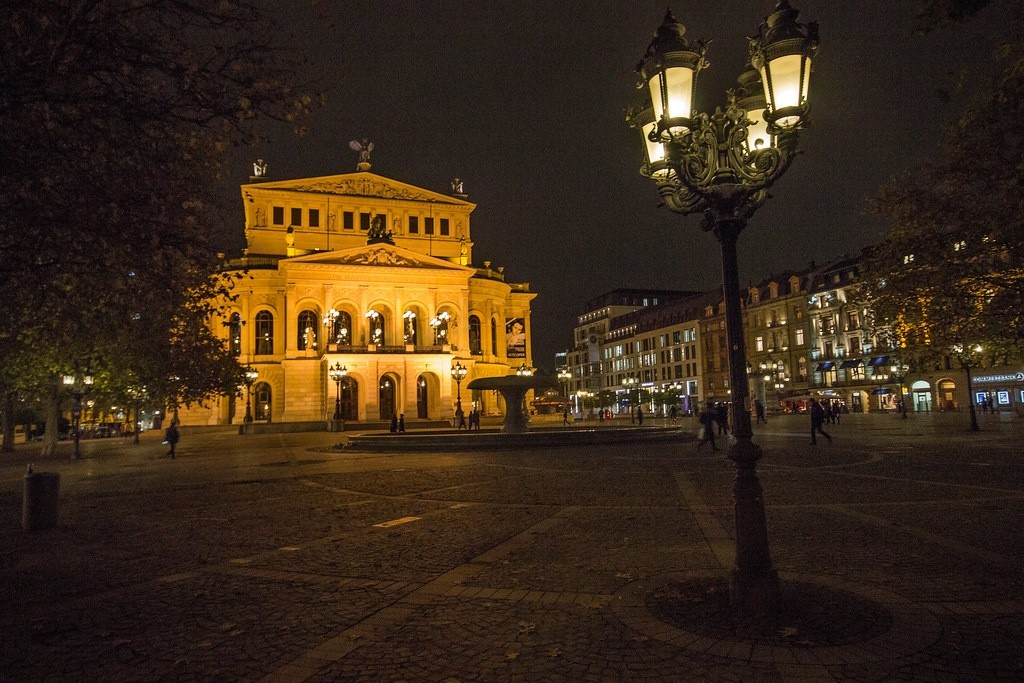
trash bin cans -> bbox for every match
[22,473,61,529]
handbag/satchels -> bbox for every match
[698,427,705,440]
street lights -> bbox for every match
[329,361,348,419]
[889,363,909,420]
[62,367,95,461]
[621,1,825,609]
[515,362,533,417]
[238,364,259,422]
[450,360,467,417]
[952,342,986,432]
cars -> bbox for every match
[12,420,125,443]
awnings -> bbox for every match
[867,355,890,366]
[871,388,892,395]
[816,363,835,371]
[838,359,862,369]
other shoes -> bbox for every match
[712,447,720,451]
[809,442,817,445]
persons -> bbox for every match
[755,400,767,424]
[820,401,840,425]
[563,410,570,426]
[459,410,480,430]
[599,408,613,422]
[988,396,994,414]
[792,402,801,415]
[390,413,397,433]
[120,422,133,438]
[163,423,179,458]
[898,400,901,413]
[506,321,525,347]
[399,414,405,432]
[668,405,697,422]
[982,398,988,414]
[808,398,832,444]
[697,401,720,452]
[638,407,643,425]
[716,402,734,435]
